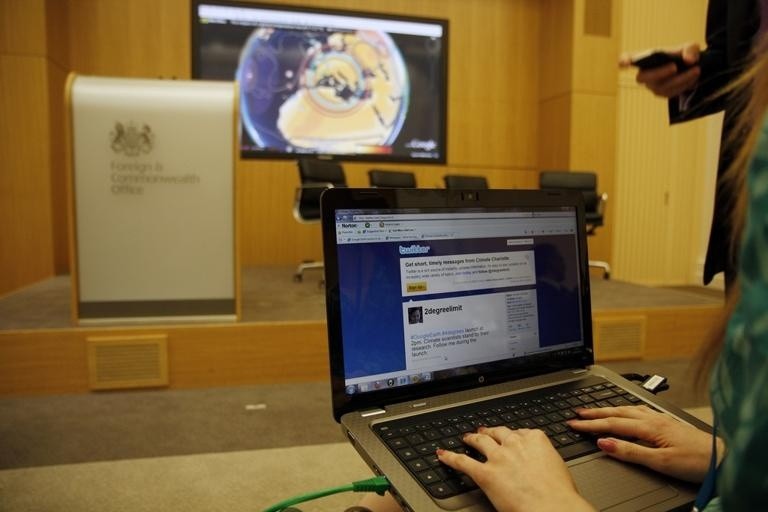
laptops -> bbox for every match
[319,186,722,512]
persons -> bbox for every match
[432,6,768,512]
[614,1,767,299]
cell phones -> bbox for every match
[627,47,696,76]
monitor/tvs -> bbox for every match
[191,0,449,164]
